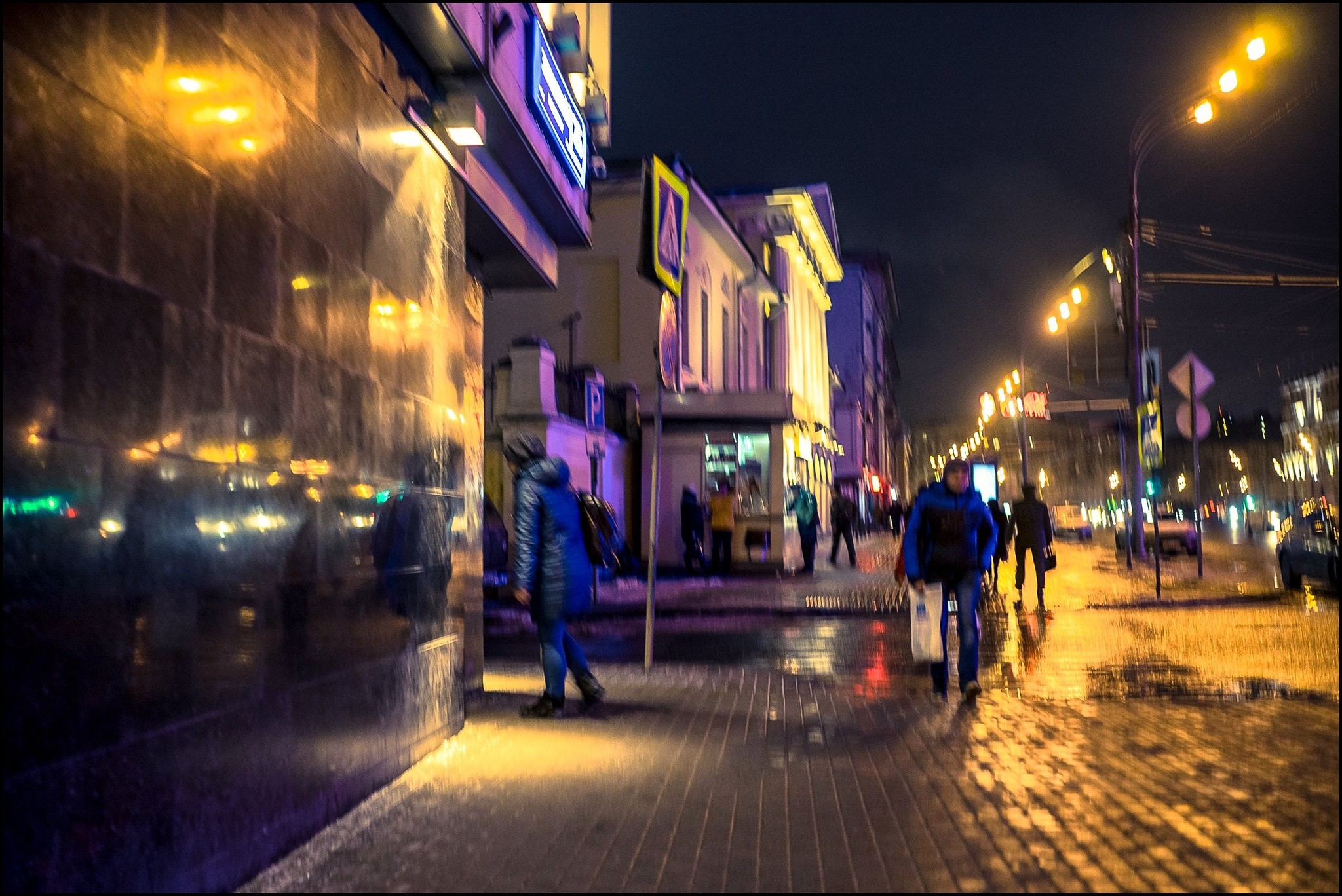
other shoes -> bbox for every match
[518,690,565,718]
[1037,588,1046,605]
[794,569,813,576]
[957,680,982,707]
[575,672,606,712]
[931,691,943,703]
[1015,582,1022,589]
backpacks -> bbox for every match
[836,498,851,531]
[568,480,639,577]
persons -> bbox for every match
[498,428,607,717]
[788,483,1053,596]
[904,459,1000,700]
[680,476,738,576]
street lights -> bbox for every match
[1020,317,1060,484]
[1131,100,1214,557]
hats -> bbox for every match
[943,459,970,479]
[502,433,547,466]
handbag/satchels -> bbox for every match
[1000,541,1010,562]
[907,582,943,664]
[1045,544,1056,572]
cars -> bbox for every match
[1275,512,1340,595]
[1114,509,1201,561]
[1049,504,1092,538]
